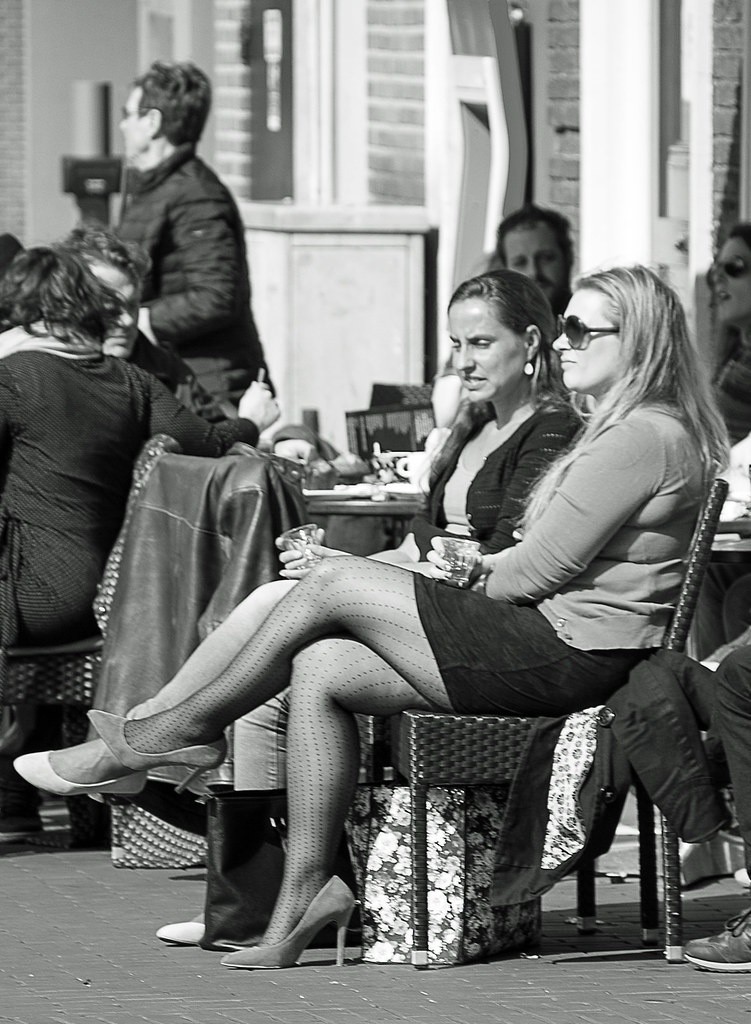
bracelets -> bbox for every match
[476,570,492,596]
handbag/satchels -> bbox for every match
[198,785,289,953]
[343,781,543,964]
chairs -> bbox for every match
[0,435,212,870]
[372,475,730,969]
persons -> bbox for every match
[108,60,275,423]
[0,205,750,972]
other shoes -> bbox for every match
[155,921,207,946]
[0,816,45,841]
[13,749,149,798]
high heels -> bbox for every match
[219,874,354,969]
[86,708,229,794]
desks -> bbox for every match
[308,499,750,560]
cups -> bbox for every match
[442,537,481,583]
[280,523,322,570]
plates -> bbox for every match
[302,483,423,502]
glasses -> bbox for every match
[555,313,619,349]
[119,103,146,117]
[711,255,747,278]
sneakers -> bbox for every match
[682,908,751,974]
